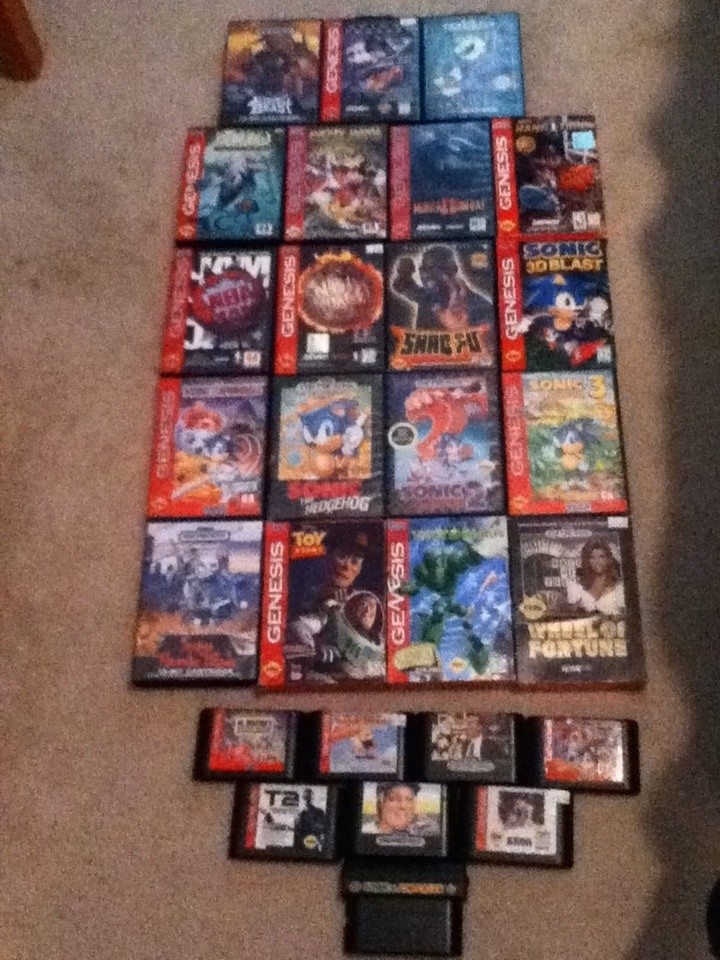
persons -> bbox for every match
[293,788,326,851]
[575,538,626,617]
[365,780,436,837]
[456,714,502,762]
[340,41,405,117]
[284,526,367,664]
